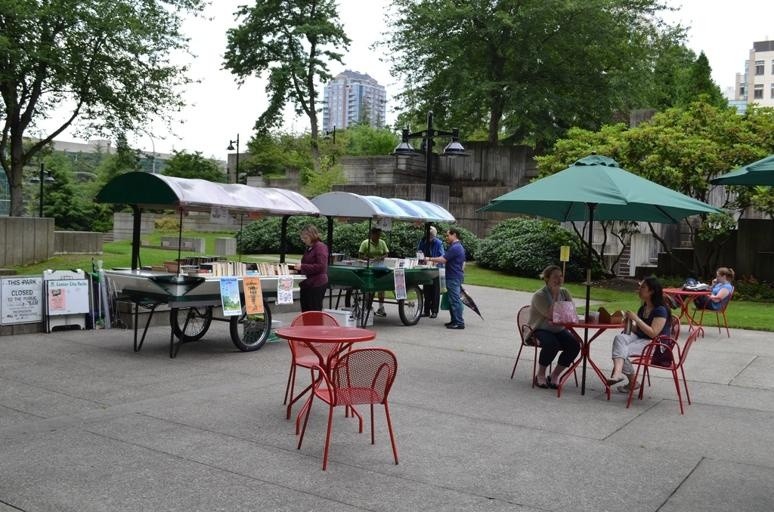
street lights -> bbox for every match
[387,111,470,256]
[29,162,57,218]
[126,126,155,174]
[226,132,241,185]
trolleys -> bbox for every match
[280,185,459,330]
[107,173,320,358]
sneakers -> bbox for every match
[617,382,642,393]
[376,310,386,317]
[664,293,678,311]
[606,375,625,384]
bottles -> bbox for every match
[625,310,631,335]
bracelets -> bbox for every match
[637,319,642,325]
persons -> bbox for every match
[417,227,445,317]
[665,266,734,310]
[525,265,580,388]
[358,226,389,317]
[293,225,328,346]
[607,276,672,392]
[424,227,465,329]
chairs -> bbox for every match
[510,303,579,390]
[604,312,681,399]
[294,346,401,471]
[688,282,736,339]
[626,323,703,414]
[282,309,356,421]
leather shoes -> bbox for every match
[420,311,438,318]
[547,373,559,389]
[444,321,465,329]
[534,374,548,389]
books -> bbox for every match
[330,253,436,270]
[143,256,293,277]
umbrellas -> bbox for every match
[476,150,727,394]
[708,155,774,184]
[460,285,483,320]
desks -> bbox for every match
[661,286,713,342]
[273,323,375,437]
[556,317,630,403]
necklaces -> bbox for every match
[643,308,650,318]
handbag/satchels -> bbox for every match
[552,300,578,325]
[650,341,673,367]
